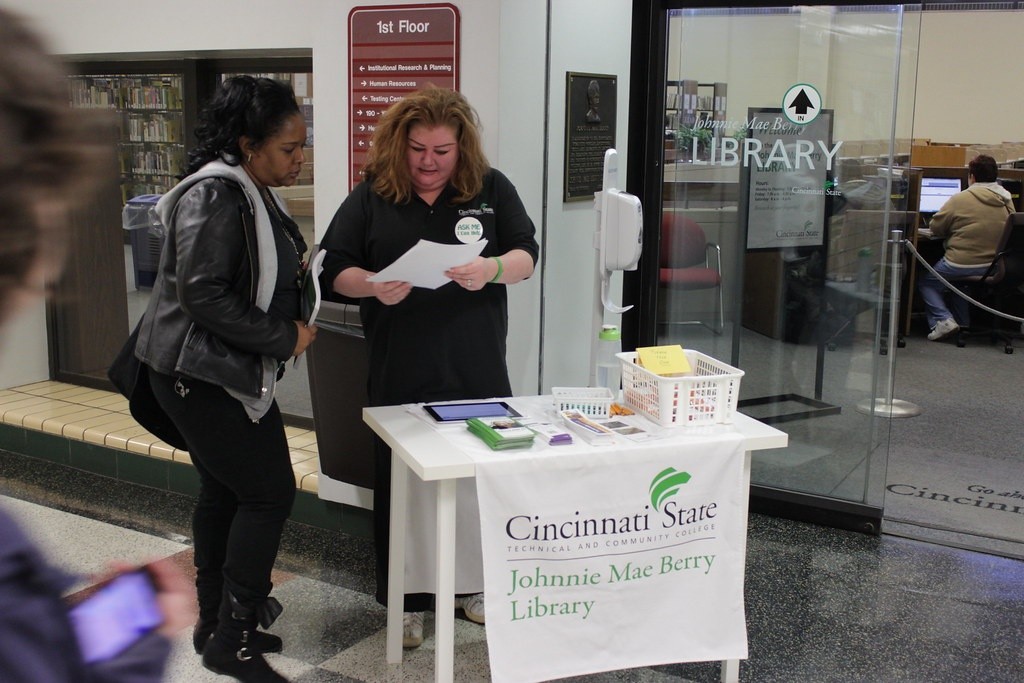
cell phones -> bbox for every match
[68,565,166,664]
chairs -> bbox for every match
[953,215,1023,354]
[661,210,726,335]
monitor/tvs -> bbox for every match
[919,176,963,216]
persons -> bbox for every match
[0,8,196,683]
[318,90,538,645]
[491,420,522,429]
[918,155,1015,341]
[587,81,601,122]
[138,75,316,683]
[789,158,902,344]
[664,117,676,139]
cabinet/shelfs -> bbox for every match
[63,70,190,208]
[665,81,727,149]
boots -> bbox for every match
[192,570,283,655]
[202,588,289,683]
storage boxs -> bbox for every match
[613,343,745,428]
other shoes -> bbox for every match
[927,318,959,340]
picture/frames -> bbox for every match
[565,72,616,203]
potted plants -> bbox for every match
[672,124,712,161]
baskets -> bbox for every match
[613,348,745,429]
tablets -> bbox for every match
[423,401,525,424]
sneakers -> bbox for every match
[455,593,485,623]
[403,613,425,647]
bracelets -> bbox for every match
[488,257,504,283]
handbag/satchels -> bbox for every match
[106,314,188,453]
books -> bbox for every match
[64,74,313,203]
[664,92,713,129]
[464,415,534,451]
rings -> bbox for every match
[467,279,473,288]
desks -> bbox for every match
[363,393,789,683]
